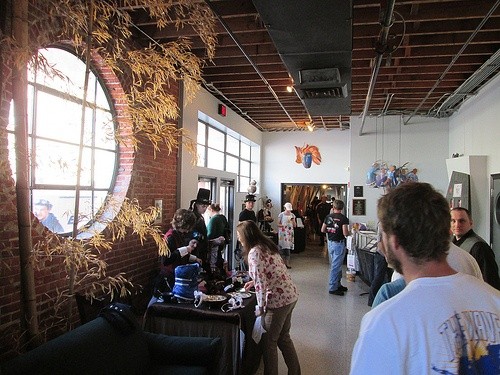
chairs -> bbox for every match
[73,280,137,326]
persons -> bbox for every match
[234,219,302,375]
[348,180,500,375]
[237,194,257,224]
[158,207,204,298]
[318,197,331,247]
[189,189,211,262]
[277,202,297,270]
[34,199,65,234]
[205,203,232,271]
[257,194,276,237]
[320,199,350,296]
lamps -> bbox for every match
[305,120,316,132]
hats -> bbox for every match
[191,188,211,205]
[244,195,256,202]
[284,203,293,210]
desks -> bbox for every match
[148,276,262,375]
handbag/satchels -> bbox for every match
[252,305,266,343]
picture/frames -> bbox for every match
[153,198,164,226]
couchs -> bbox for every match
[0,302,222,375]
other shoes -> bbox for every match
[338,285,348,292]
[329,289,344,296]
[286,265,292,269]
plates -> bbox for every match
[202,295,227,302]
[235,288,245,292]
[227,292,252,298]
